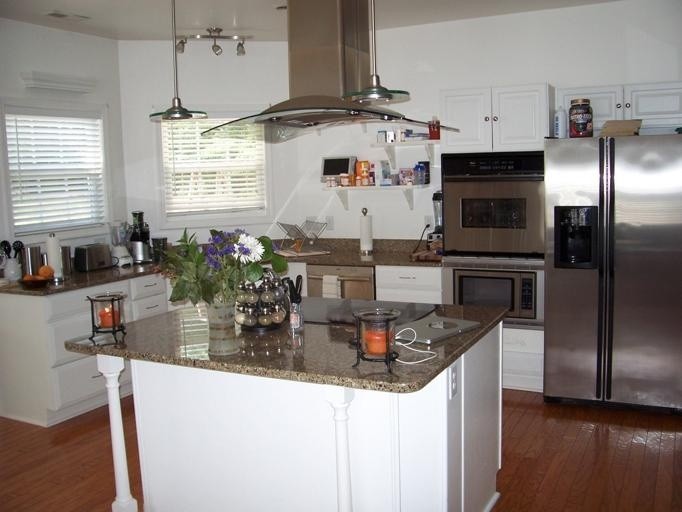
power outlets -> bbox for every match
[424,215,434,231]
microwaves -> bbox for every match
[443,266,545,332]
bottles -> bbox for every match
[236,274,305,329]
[48,232,63,278]
[236,330,304,366]
[5,259,22,281]
[427,116,441,140]
[325,160,430,185]
[554,105,569,138]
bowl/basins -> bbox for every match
[20,280,51,288]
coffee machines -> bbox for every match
[130,212,152,265]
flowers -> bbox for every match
[158,220,291,310]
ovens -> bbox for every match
[303,263,376,302]
[442,153,543,257]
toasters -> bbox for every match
[75,243,112,271]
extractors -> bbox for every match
[200,1,461,147]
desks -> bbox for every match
[67,295,514,512]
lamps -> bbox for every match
[143,2,211,123]
[341,1,415,118]
[359,206,375,260]
[173,24,254,56]
[82,287,129,349]
[349,303,402,375]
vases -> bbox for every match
[204,288,243,358]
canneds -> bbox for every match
[326,161,370,187]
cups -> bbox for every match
[153,237,169,256]
[21,246,43,275]
[62,246,71,274]
[43,253,48,266]
[90,292,124,330]
[357,308,401,358]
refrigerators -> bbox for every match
[542,133,681,410]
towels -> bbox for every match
[321,274,343,300]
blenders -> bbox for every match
[428,193,443,249]
[107,219,134,269]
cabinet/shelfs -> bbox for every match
[263,258,309,298]
[549,78,682,138]
[1,278,133,432]
[373,261,444,306]
[319,136,441,211]
[439,80,556,156]
[129,271,167,322]
[166,271,194,312]
[500,324,546,396]
